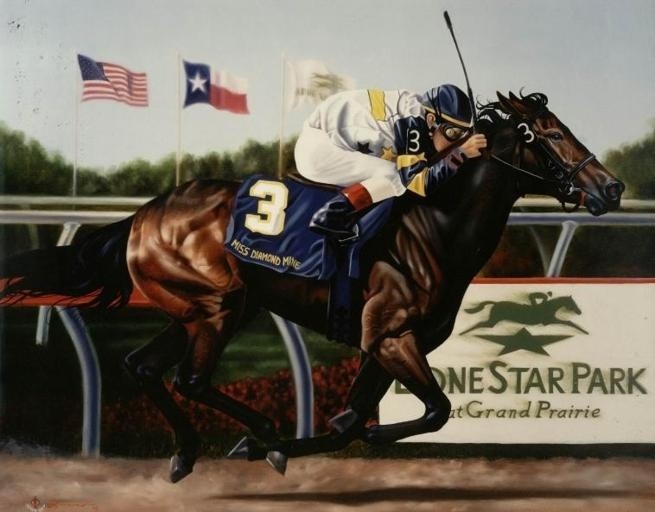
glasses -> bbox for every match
[433,119,465,142]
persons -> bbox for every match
[293,82,488,240]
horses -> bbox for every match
[1,85,627,486]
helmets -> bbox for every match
[421,83,474,129]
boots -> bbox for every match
[308,192,355,236]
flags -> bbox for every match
[75,52,150,108]
[180,58,251,116]
[284,53,354,113]
